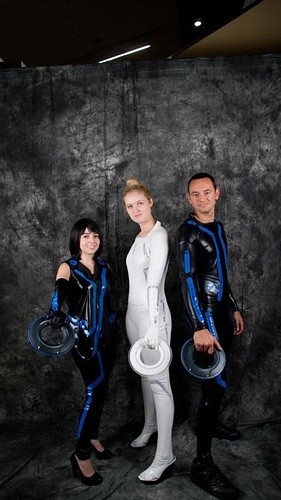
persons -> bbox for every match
[49,216,117,485]
[173,172,245,500]
[124,179,177,485]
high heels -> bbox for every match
[90,442,113,460]
[130,425,157,448]
[138,456,176,481]
[70,452,103,486]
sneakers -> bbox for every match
[190,456,241,500]
[193,420,241,441]
